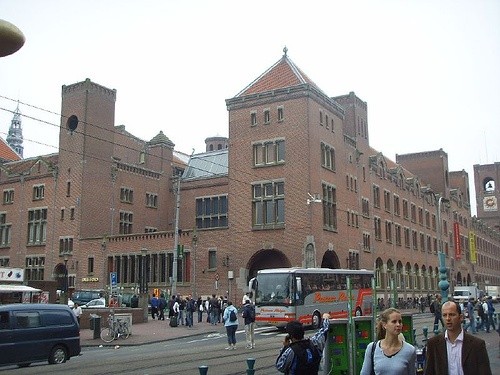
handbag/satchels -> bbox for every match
[199,304,203,311]
[179,304,184,310]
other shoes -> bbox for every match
[245,346,252,349]
[225,346,233,349]
[232,346,236,349]
[252,345,255,348]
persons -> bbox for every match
[73,303,82,329]
[110,293,256,351]
[275,294,496,375]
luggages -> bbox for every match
[170,313,177,326]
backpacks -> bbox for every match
[478,303,485,316]
[230,310,237,321]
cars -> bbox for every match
[79,299,105,307]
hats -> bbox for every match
[286,320,304,339]
[243,300,250,305]
[227,302,232,304]
[486,296,492,299]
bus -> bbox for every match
[249,268,374,331]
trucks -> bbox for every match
[452,286,500,306]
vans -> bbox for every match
[72,291,101,306]
[0,304,83,368]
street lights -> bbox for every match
[62,253,68,294]
[420,186,450,303]
[140,248,148,293]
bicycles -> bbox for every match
[100,312,131,343]
